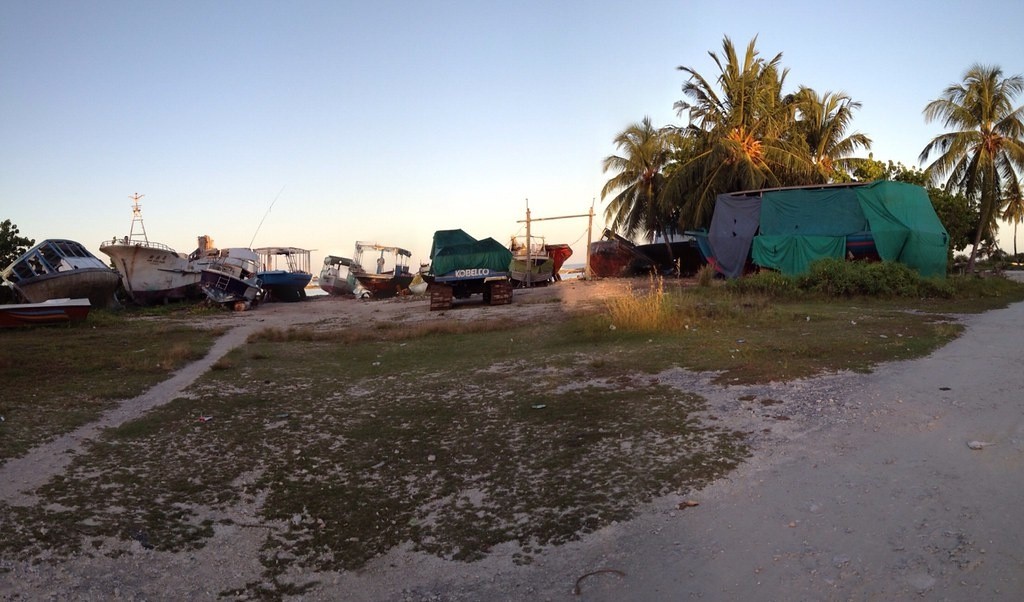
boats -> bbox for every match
[319,257,358,295]
[100,190,203,303]
[350,240,428,295]
[590,228,640,279]
[0,238,120,304]
[188,249,263,304]
[255,248,315,297]
[507,234,553,283]
[0,298,91,328]
[545,244,574,278]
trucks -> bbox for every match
[419,227,513,311]
